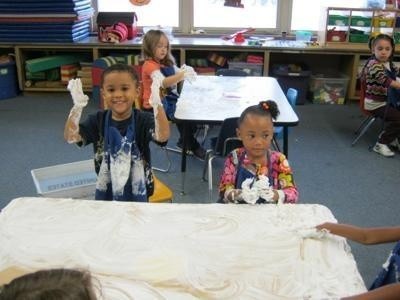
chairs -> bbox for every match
[351,77,400,150]
[130,66,298,203]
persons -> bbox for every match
[63,63,172,202]
[217,99,302,205]
[0,266,99,299]
[359,33,400,157]
[313,221,400,299]
[137,29,209,162]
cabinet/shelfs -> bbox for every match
[0,36,400,99]
[321,6,400,44]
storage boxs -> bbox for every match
[327,15,400,44]
[273,63,311,105]
[227,60,264,76]
[310,72,351,105]
[0,60,21,99]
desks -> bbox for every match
[0,196,369,300]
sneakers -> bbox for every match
[176,137,207,160]
[372,141,395,157]
